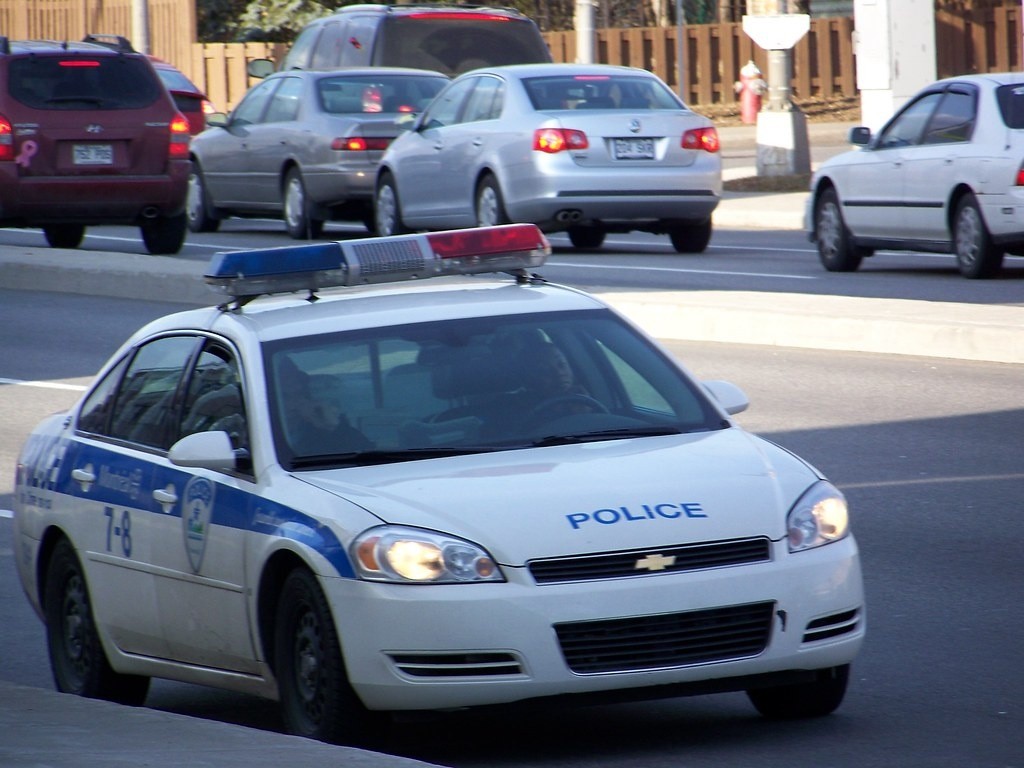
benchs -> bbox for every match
[333,363,435,451]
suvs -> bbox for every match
[0,32,189,257]
[242,4,554,82]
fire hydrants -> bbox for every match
[732,61,767,124]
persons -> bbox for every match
[237,358,376,469]
[533,343,580,396]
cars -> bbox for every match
[187,68,452,240]
[803,72,1024,280]
[142,53,214,139]
[373,62,724,254]
[12,222,867,746]
